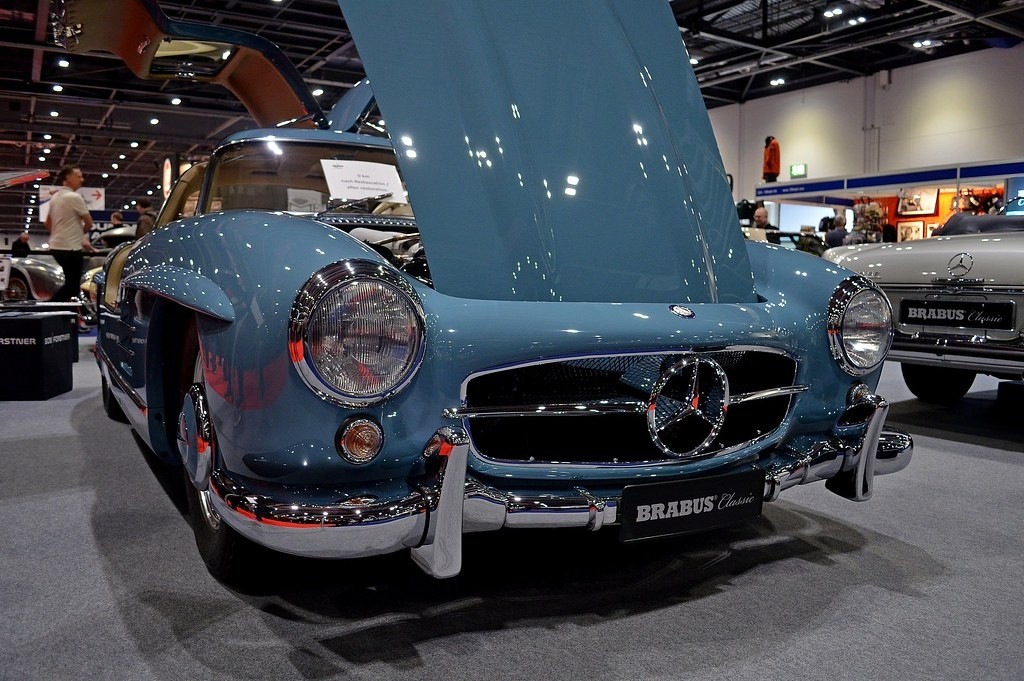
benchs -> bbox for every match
[945,215,1024,235]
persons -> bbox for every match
[853,196,884,220]
[101,212,124,248]
[11,232,31,258]
[135,197,158,240]
[45,165,99,334]
[751,207,850,248]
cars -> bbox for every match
[820,196,1024,405]
[0,0,915,593]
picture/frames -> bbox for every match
[896,221,924,243]
[896,188,939,216]
